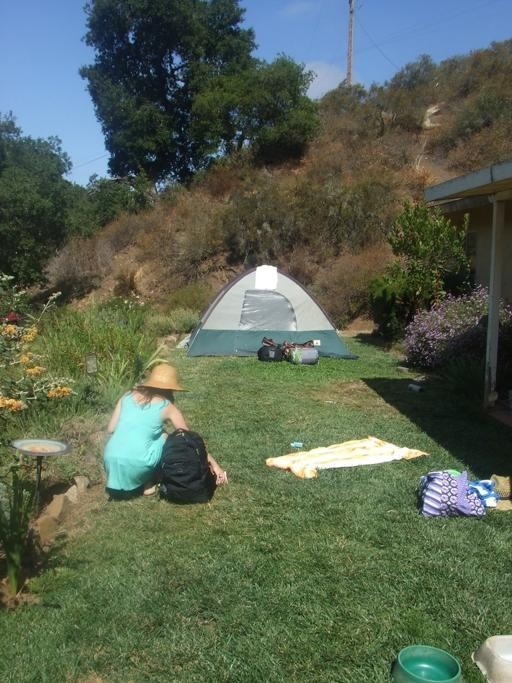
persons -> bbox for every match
[103,364,228,502]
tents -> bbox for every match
[185,263,359,359]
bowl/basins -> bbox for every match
[393,644,461,683]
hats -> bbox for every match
[137,363,191,393]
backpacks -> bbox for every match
[159,427,217,504]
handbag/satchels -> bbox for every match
[257,337,319,365]
